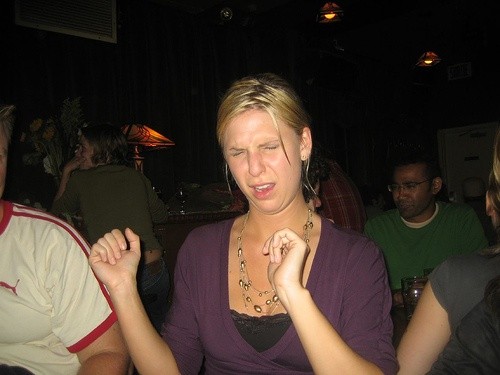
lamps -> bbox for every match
[316,1,344,24]
[199,6,234,23]
[118,124,176,174]
[415,49,441,67]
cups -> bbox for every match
[401,275,427,319]
[176,184,191,212]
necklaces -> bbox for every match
[238,206,314,316]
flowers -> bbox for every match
[20,95,84,212]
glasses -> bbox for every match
[389,176,436,192]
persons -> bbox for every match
[398,134,500,375]
[88,74,397,375]
[304,142,363,233]
[0,104,131,375]
[51,120,177,302]
[365,157,486,320]
